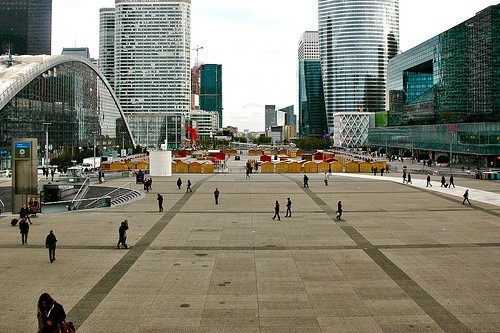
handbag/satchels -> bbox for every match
[52,320,76,333]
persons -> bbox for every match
[285,198,292,217]
[336,201,343,220]
[372,154,493,188]
[324,173,328,186]
[36,293,65,333]
[463,189,471,205]
[272,201,281,220]
[186,180,192,193]
[303,175,309,188]
[18,165,164,262]
[214,188,219,204]
[246,168,250,178]
[177,178,183,189]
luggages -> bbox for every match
[11,216,21,226]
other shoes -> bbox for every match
[51,260,53,263]
[337,216,338,219]
[339,219,341,220]
[289,216,291,217]
[285,216,287,217]
[25,241,27,244]
[117,246,120,249]
[30,223,32,225]
[53,258,55,260]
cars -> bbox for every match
[115,154,139,162]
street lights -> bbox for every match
[92,132,98,170]
[43,123,51,169]
[121,132,126,160]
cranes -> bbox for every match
[191,45,203,68]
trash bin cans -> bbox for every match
[105,197,111,206]
[235,155,240,160]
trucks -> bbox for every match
[82,157,100,169]
